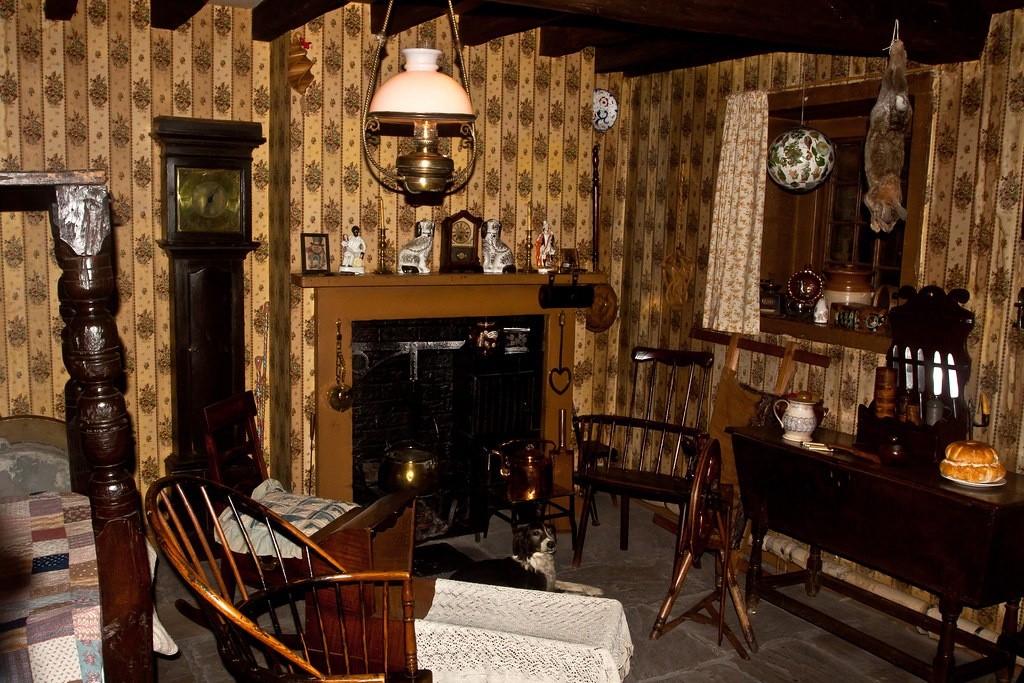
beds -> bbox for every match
[0,414,105,683]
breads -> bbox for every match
[938,440,1005,484]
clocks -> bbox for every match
[176,166,244,233]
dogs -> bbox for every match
[481,219,513,274]
[398,219,435,273]
[450,523,604,597]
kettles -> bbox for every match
[491,439,557,504]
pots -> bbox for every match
[384,443,440,493]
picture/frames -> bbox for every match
[301,233,331,276]
[440,210,483,275]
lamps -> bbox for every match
[368,0,477,198]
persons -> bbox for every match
[535,221,555,274]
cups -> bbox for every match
[926,395,953,427]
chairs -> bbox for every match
[147,474,433,682]
[201,391,363,603]
[568,345,715,565]
[887,284,974,438]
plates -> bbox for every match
[940,473,1007,488]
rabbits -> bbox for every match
[863,37,912,234]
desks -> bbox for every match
[728,417,1023,683]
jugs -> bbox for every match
[773,399,817,442]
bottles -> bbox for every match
[822,265,876,310]
[813,298,829,324]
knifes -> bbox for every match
[892,345,959,420]
